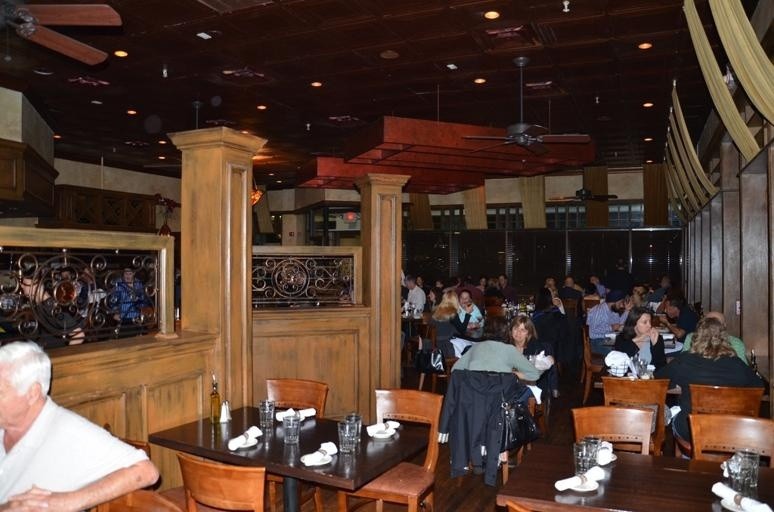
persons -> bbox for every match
[1,341,160,512]
[400,266,770,474]
[1,258,150,345]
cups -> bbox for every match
[343,412,362,443]
[581,438,599,466]
[573,441,593,475]
[282,416,300,444]
[336,420,357,452]
[726,458,753,498]
[734,447,760,487]
[258,399,274,428]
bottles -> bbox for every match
[224,400,232,420]
[209,381,220,424]
[219,400,229,423]
[518,296,526,317]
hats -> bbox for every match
[606,289,625,303]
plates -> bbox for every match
[299,453,331,466]
[276,411,305,421]
[720,498,738,511]
[569,481,599,492]
[610,452,617,461]
[238,438,257,448]
[366,424,395,439]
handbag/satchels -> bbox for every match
[413,348,446,374]
[499,402,539,453]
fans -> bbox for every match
[462,56,590,154]
[0,0,122,67]
[546,164,619,204]
